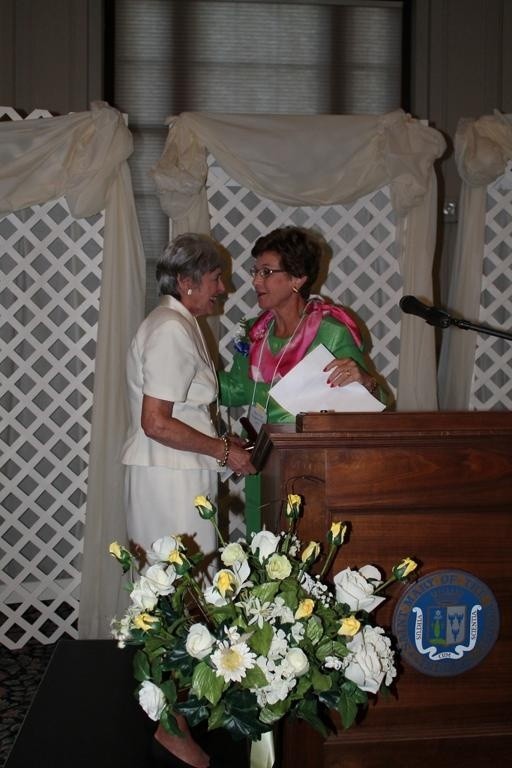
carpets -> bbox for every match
[0,637,59,768]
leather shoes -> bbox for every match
[152,736,216,768]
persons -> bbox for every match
[218,228,391,545]
[114,233,257,767]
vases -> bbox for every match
[250,731,275,767]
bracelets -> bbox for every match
[220,435,228,468]
[368,376,379,395]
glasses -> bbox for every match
[251,266,286,278]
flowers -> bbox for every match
[107,491,420,768]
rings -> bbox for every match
[236,472,242,478]
[345,371,350,376]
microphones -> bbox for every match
[398,292,453,328]
